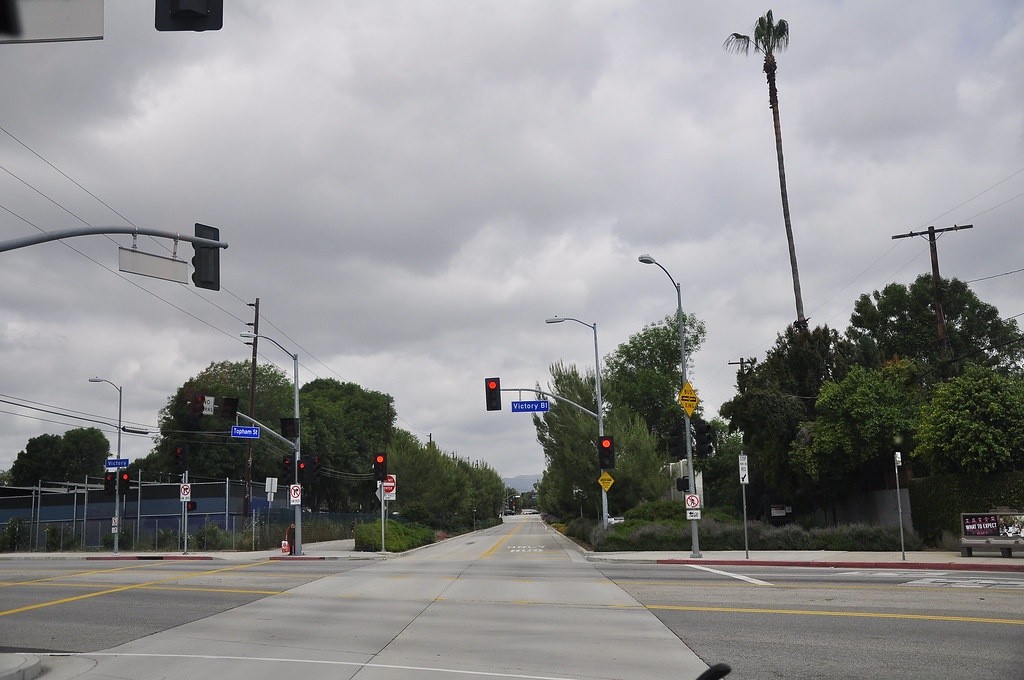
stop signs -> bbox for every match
[384,476,395,493]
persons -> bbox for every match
[285,521,296,555]
[999,517,1021,536]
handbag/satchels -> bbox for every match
[281,541,290,553]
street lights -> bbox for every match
[638,254,704,559]
[240,332,303,558]
[88,378,123,554]
[545,317,609,531]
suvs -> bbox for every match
[504,510,516,516]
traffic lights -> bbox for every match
[283,456,294,478]
[374,452,387,481]
[484,378,502,411]
[195,394,205,419]
[188,502,196,511]
[696,420,713,458]
[176,447,183,455]
[119,471,130,494]
[597,436,616,470]
[104,472,116,494]
[667,422,684,457]
[296,460,306,484]
